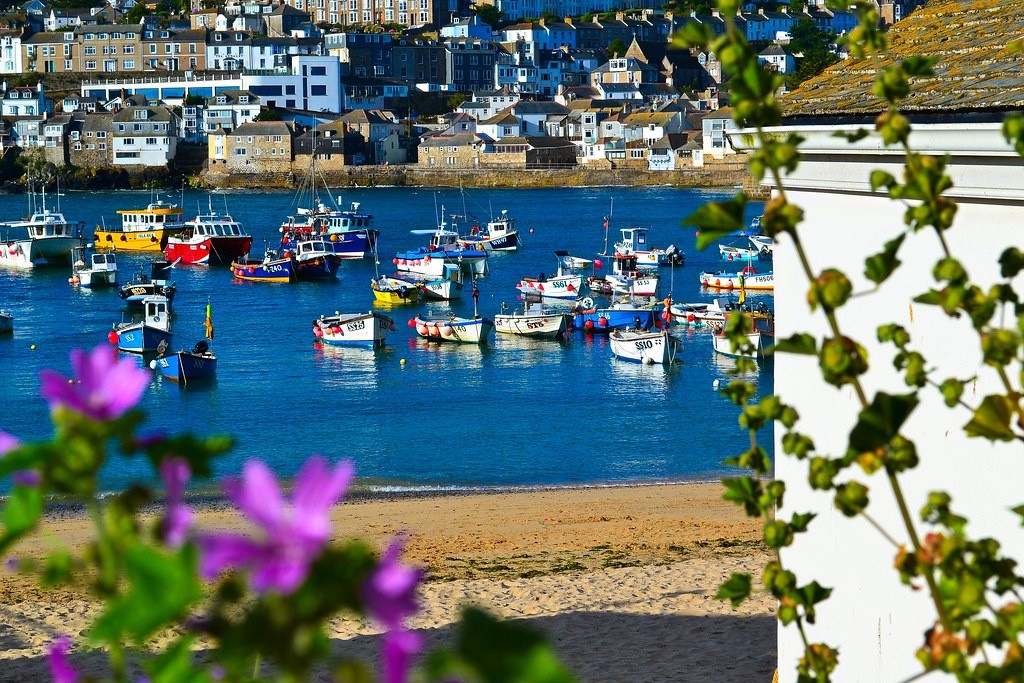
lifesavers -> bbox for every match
[742,267,755,275]
[470,225,480,236]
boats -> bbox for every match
[312,310,392,350]
[1,164,86,271]
[711,322,764,366]
[410,289,492,349]
[149,295,219,387]
[110,292,174,357]
[117,254,182,300]
[607,328,686,367]
[158,191,253,266]
[234,113,773,340]
[71,243,122,291]
[92,181,198,256]
